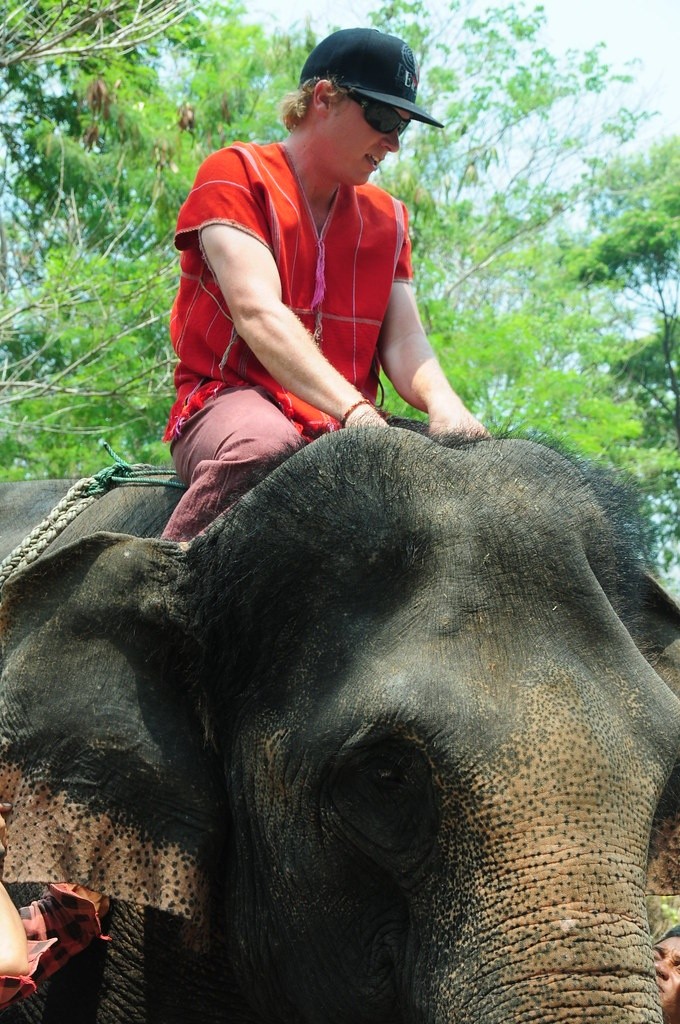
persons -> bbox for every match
[160,27,491,542]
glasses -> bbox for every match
[345,87,411,137]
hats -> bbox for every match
[300,27,444,129]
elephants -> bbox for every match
[2,420,680,1024]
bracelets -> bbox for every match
[341,399,379,428]
